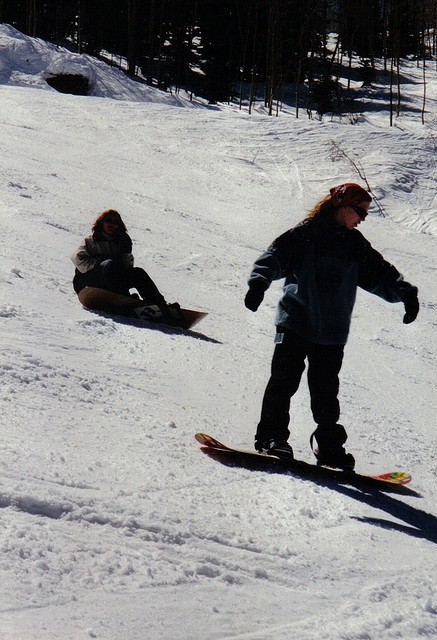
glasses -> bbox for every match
[348,203,367,218]
[102,218,119,224]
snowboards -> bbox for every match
[79,287,209,330]
[194,433,412,484]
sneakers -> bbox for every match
[133,304,164,320]
[258,432,293,455]
[166,302,187,328]
[317,447,354,468]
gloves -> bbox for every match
[98,259,115,277]
[244,288,264,312]
[400,286,419,324]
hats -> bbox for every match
[330,183,372,207]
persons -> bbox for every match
[244,182,420,469]
[70,210,181,307]
